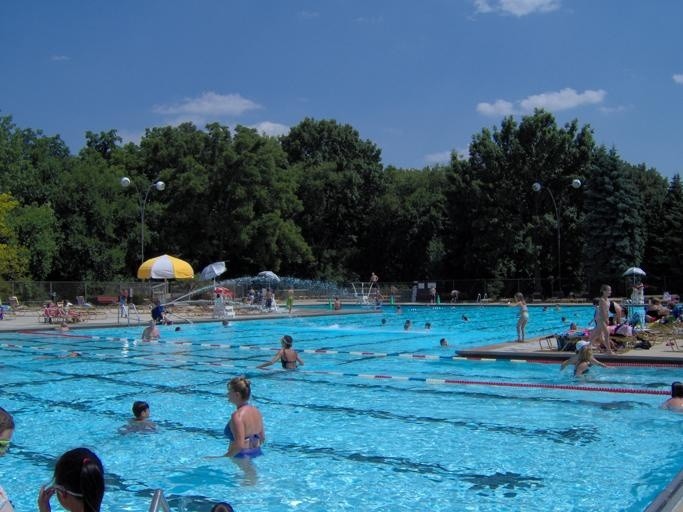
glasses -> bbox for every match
[0,440,10,451]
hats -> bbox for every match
[575,340,590,351]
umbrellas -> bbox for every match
[620,267,645,287]
[136,254,195,304]
[256,271,278,286]
[200,260,224,290]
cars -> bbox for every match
[190,281,233,300]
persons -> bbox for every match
[390,285,401,312]
[120,402,156,437]
[562,317,576,332]
[450,290,460,303]
[140,320,160,339]
[45,292,70,332]
[36,448,104,510]
[335,297,341,310]
[118,289,127,318]
[507,292,529,342]
[644,296,683,323]
[380,315,469,347]
[560,341,607,377]
[230,452,260,487]
[151,306,182,332]
[543,304,559,310]
[588,284,622,355]
[371,273,384,310]
[0,407,15,511]
[663,382,682,410]
[256,335,305,369]
[205,375,264,456]
[214,287,294,325]
[430,287,437,304]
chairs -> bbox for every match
[538,291,682,354]
[31,282,110,329]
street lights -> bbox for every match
[120,177,164,283]
[532,179,582,292]
